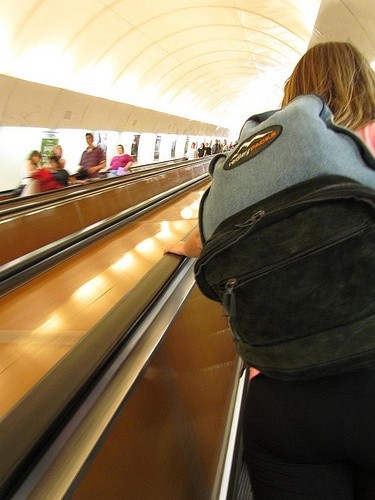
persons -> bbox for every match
[188,137,237,159]
[163,42,375,500]
[19,133,134,198]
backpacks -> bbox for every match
[194,95,375,379]
